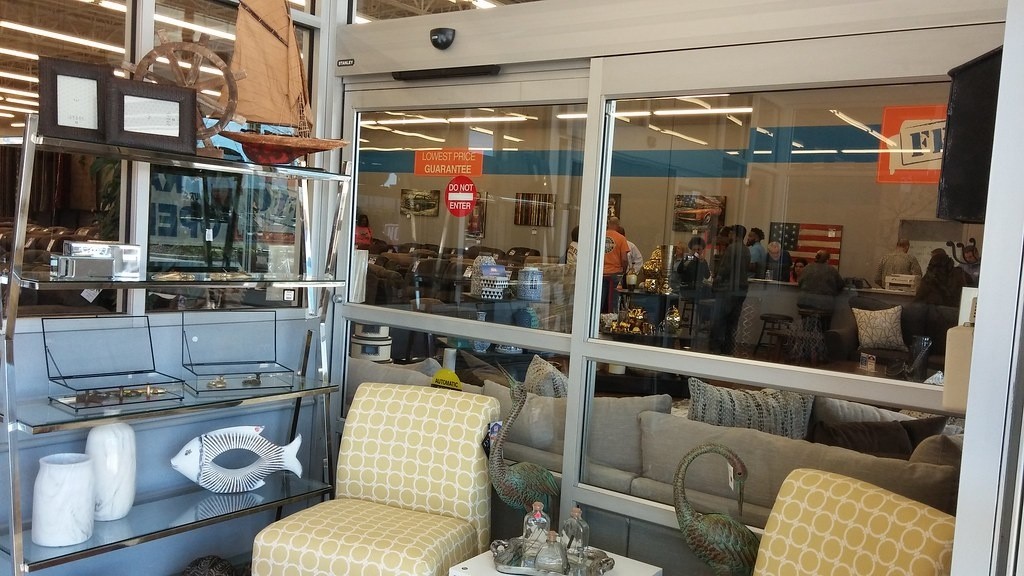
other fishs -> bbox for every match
[171,425,303,493]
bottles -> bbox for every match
[30,452,93,547]
[765,270,771,280]
[85,408,136,522]
[533,531,570,575]
[560,507,590,572]
[517,267,543,301]
[521,502,550,568]
[470,252,497,297]
[472,311,491,353]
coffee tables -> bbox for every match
[449,545,663,576]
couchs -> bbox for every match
[347,355,963,529]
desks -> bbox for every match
[617,288,678,324]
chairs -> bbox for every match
[367,244,540,305]
[251,383,501,576]
[751,469,955,576]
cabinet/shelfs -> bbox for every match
[1,113,351,576]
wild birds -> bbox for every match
[674,444,759,576]
[488,361,559,514]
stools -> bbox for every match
[753,314,794,364]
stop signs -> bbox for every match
[444,177,478,217]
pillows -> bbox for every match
[851,305,909,353]
[446,348,948,460]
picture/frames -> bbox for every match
[105,76,198,154]
[38,53,113,141]
[465,192,488,240]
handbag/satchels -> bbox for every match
[885,334,932,383]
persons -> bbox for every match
[708,225,752,355]
[566,225,579,288]
[368,263,403,280]
[875,238,923,290]
[355,214,373,254]
[917,243,981,305]
[797,250,843,330]
[601,217,643,315]
[670,225,807,302]
